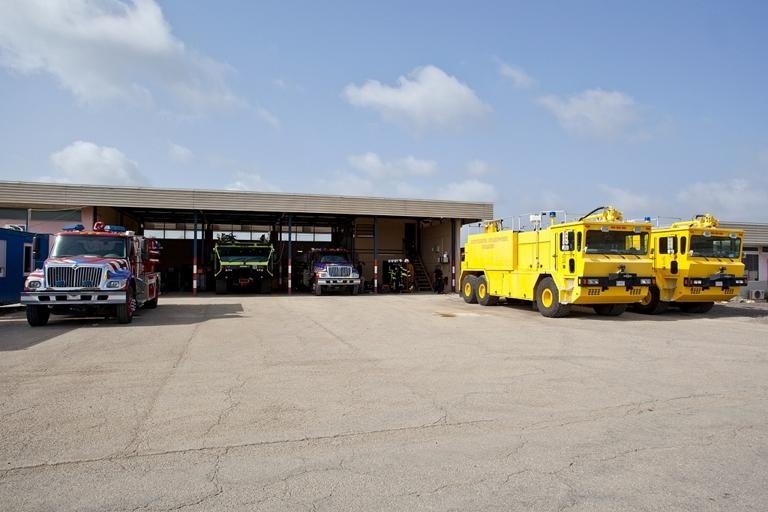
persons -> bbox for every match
[404,259,414,277]
[431,265,443,294]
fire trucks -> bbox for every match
[0,224,162,327]
[212,238,277,294]
[458,204,748,318]
[298,248,361,296]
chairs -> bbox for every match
[64,244,85,255]
